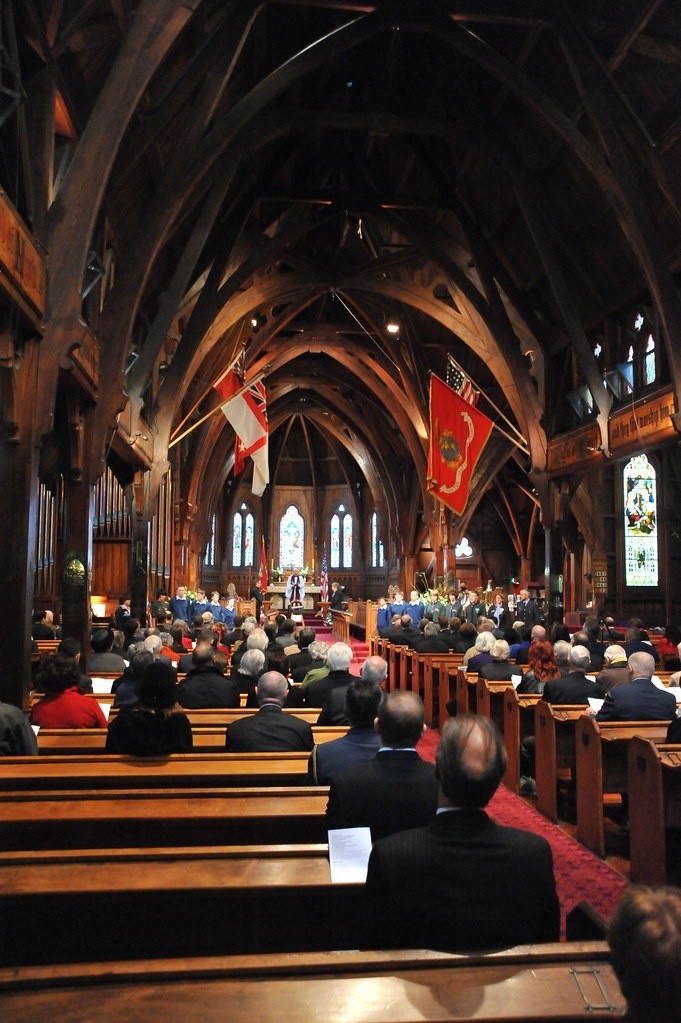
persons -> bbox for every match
[326,689,437,841]
[308,679,384,785]
[0,585,388,757]
[376,589,681,831]
[285,568,305,607]
[606,885,681,1023]
[359,712,559,950]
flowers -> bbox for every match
[273,567,284,578]
[301,567,309,578]
[164,578,201,611]
[410,576,448,615]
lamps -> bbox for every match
[386,314,400,335]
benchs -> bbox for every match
[0,636,681,1023]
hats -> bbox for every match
[133,662,179,708]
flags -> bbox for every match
[321,549,328,615]
[257,543,268,595]
[214,349,249,476]
[446,359,480,407]
[427,374,494,516]
[220,380,269,496]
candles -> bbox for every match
[312,559,314,570]
[271,559,274,570]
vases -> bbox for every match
[304,576,306,582]
[279,576,281,582]
